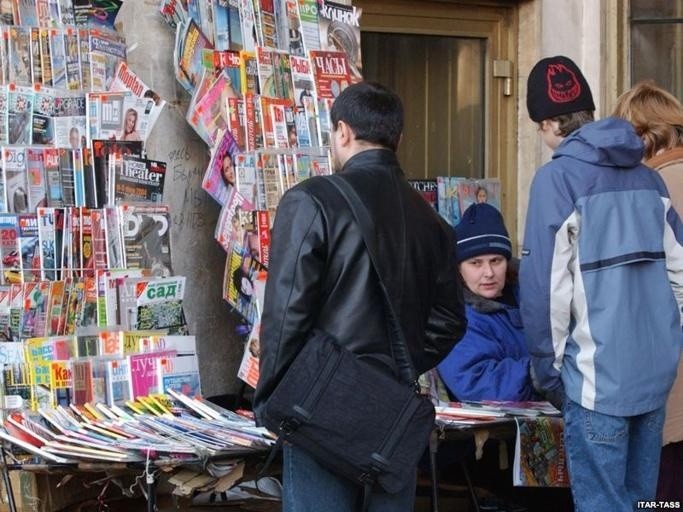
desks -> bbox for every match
[3,446,283,512]
[429,401,564,512]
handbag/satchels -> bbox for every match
[263,327,435,496]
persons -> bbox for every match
[434,199,576,512]
[514,53,682,512]
[248,78,468,511]
[612,82,683,510]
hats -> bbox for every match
[526,55,595,121]
[454,202,512,262]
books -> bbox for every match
[1,0,576,492]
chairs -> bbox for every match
[430,364,481,512]
